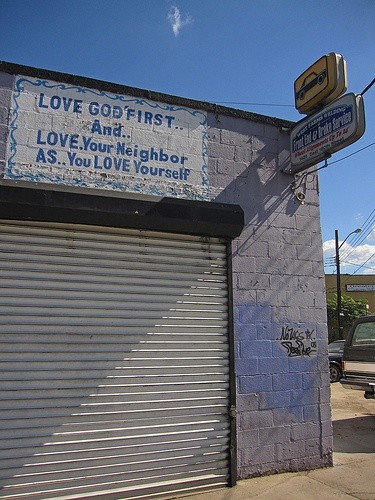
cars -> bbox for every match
[329,315,375,385]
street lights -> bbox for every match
[334,228,361,340]
